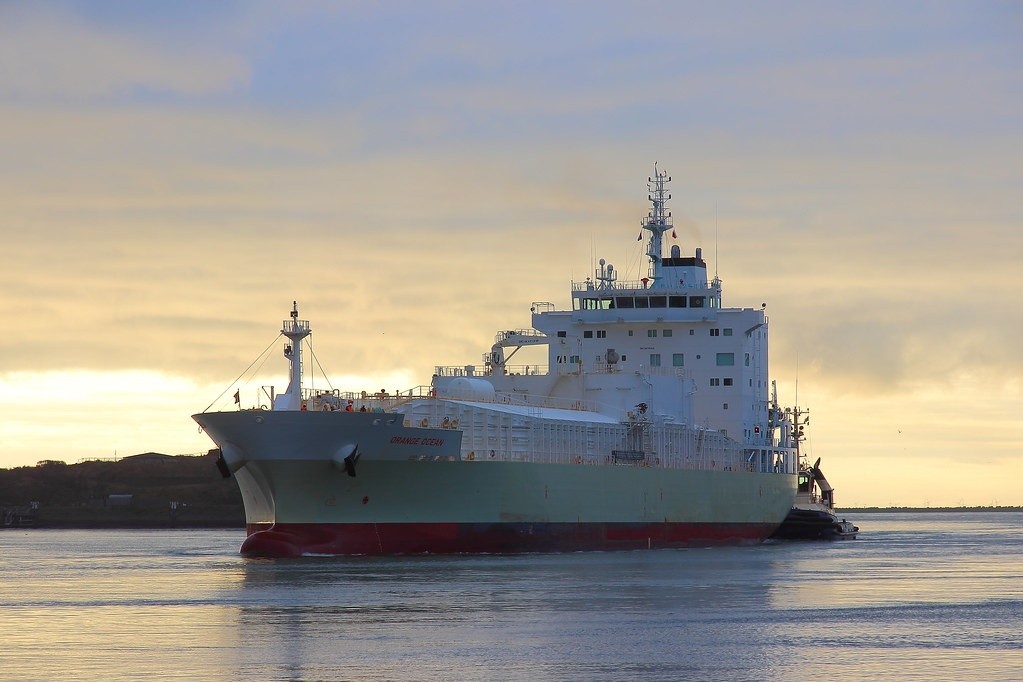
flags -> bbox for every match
[637,230,642,241]
[672,230,677,239]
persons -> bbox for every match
[322,401,367,413]
[301,404,307,411]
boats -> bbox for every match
[777,378,859,541]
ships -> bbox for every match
[191,160,798,557]
